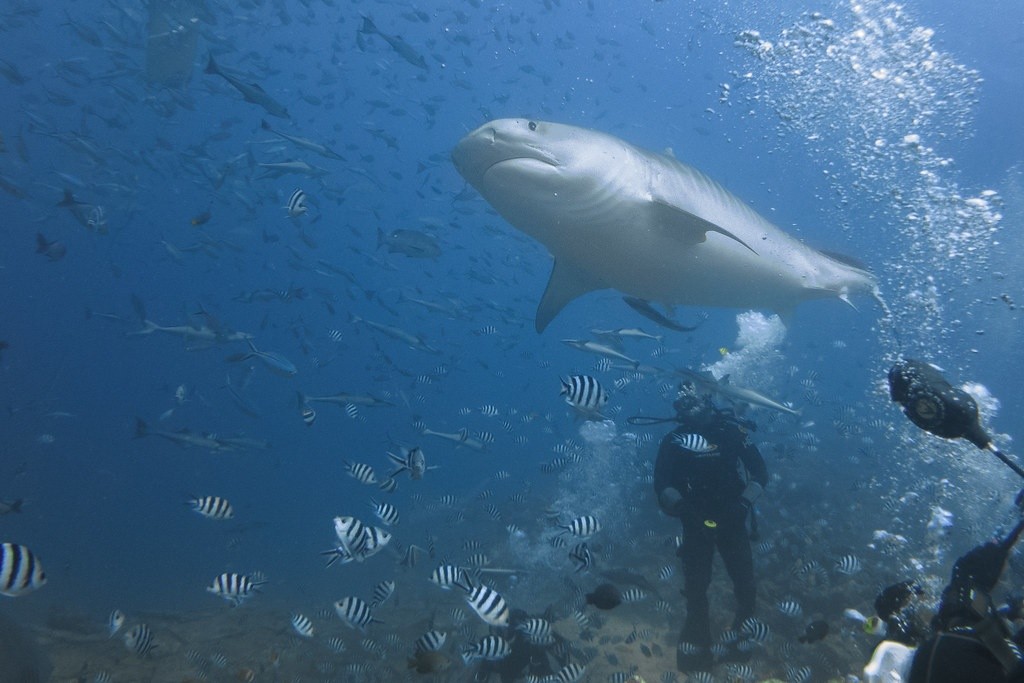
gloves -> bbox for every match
[732,496,751,519]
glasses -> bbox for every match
[674,395,698,413]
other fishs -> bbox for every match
[1,0,1023,683]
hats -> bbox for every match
[678,381,711,420]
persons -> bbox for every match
[874,517,1024,664]
[653,381,769,675]
